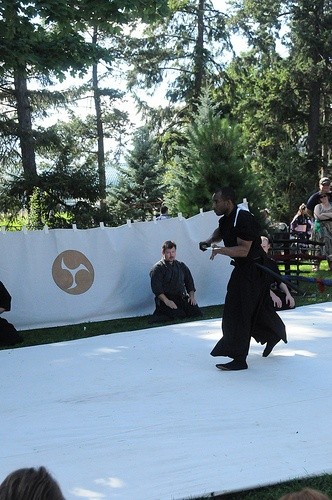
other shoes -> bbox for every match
[263,339,281,357]
[216,360,248,371]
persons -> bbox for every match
[199,187,287,370]
[260,236,295,311]
[312,190,332,272]
[0,281,23,348]
[290,203,315,263]
[149,241,203,320]
[305,177,332,222]
